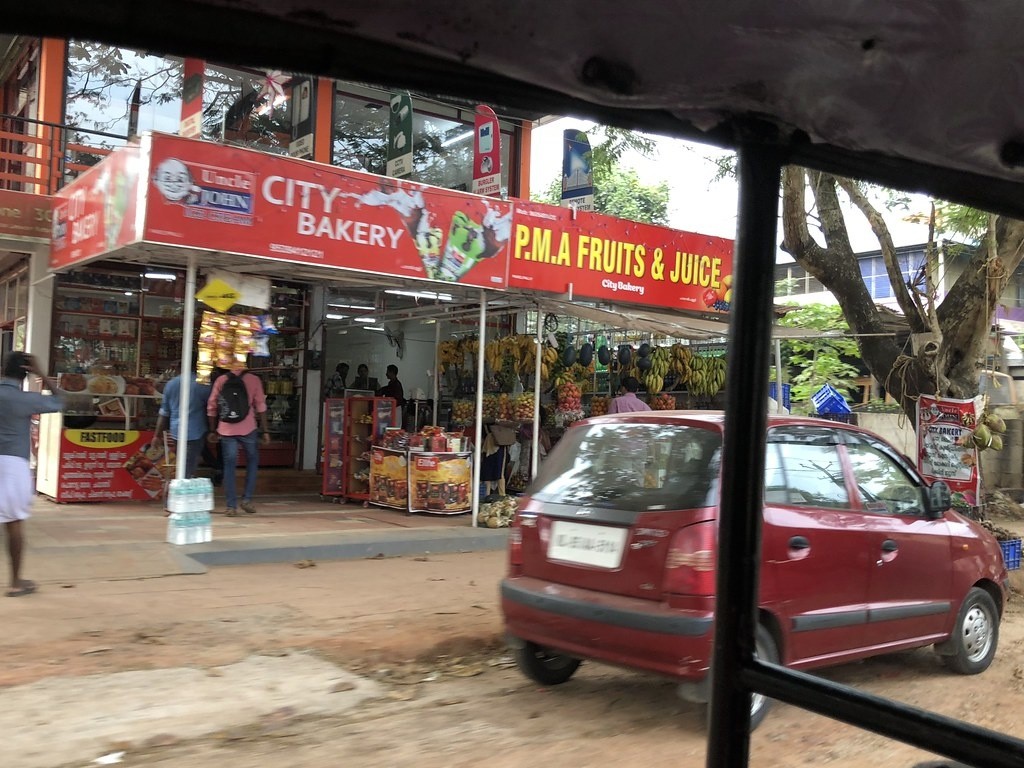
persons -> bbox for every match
[606,374,653,414]
[152,354,214,514]
[1,350,68,600]
[320,362,351,411]
[348,363,382,397]
[205,369,272,517]
[477,423,508,500]
[375,364,408,410]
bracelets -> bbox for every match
[261,430,271,434]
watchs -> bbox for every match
[208,430,216,434]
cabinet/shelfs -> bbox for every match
[347,396,400,505]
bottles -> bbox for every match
[163,474,213,549]
[96,340,138,362]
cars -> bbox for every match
[499,407,1012,735]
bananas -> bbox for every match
[438,331,727,399]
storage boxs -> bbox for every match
[807,413,859,427]
[811,383,851,415]
[768,381,791,413]
[996,537,1021,571]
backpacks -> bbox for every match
[215,368,252,424]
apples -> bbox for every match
[557,381,582,413]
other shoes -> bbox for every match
[241,498,257,513]
[5,579,38,596]
[225,507,238,517]
